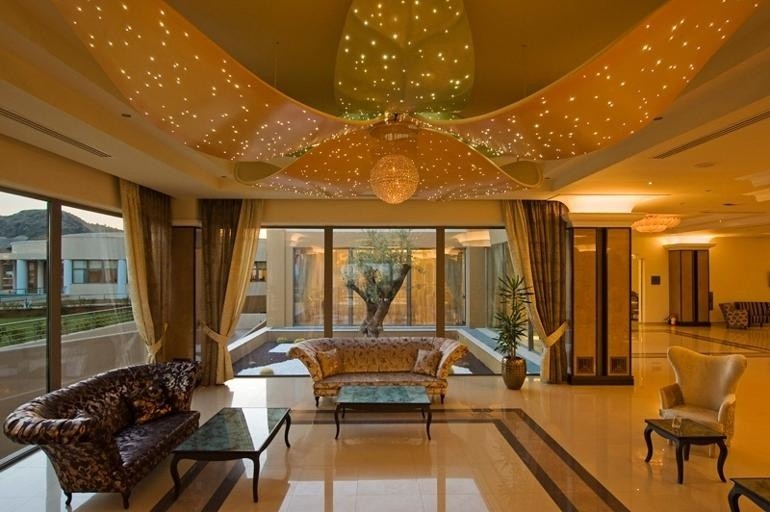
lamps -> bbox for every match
[368,119,420,203]
[628,214,683,234]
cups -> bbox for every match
[671,415,683,428]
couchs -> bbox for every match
[4,354,200,511]
[660,344,746,460]
[287,337,466,406]
[718,301,769,330]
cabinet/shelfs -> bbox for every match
[669,249,711,328]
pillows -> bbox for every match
[413,349,442,378]
[315,348,346,378]
[128,379,176,424]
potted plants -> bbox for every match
[494,274,534,390]
